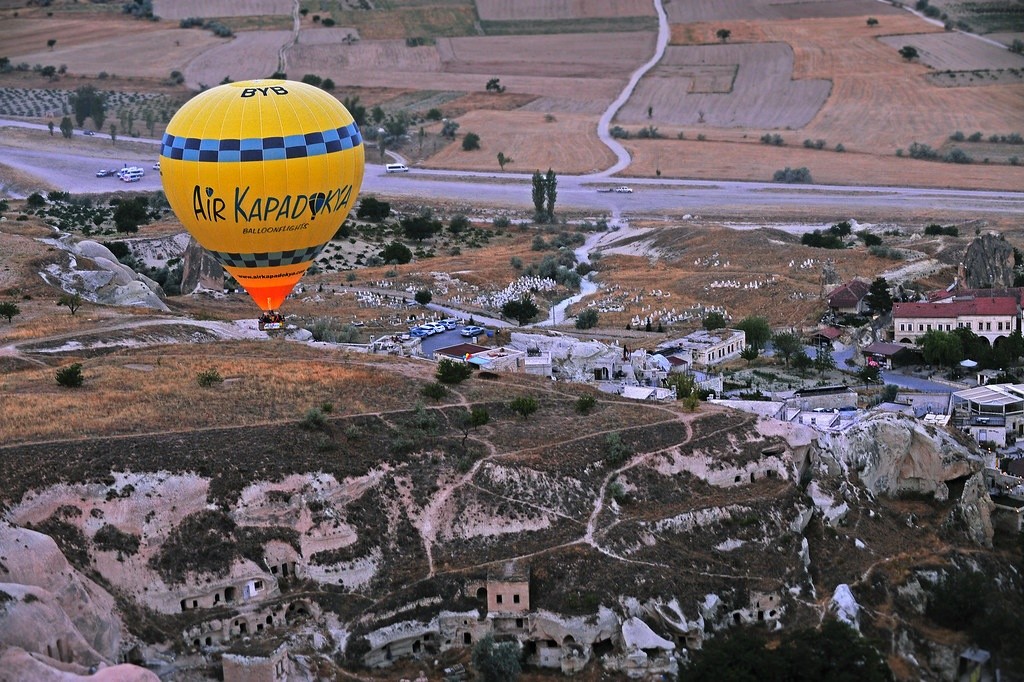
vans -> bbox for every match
[385,163,409,173]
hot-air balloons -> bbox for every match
[158,79,366,332]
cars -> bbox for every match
[83,129,96,135]
[616,186,632,194]
[96,166,144,183]
[459,326,485,337]
[405,319,456,340]
[152,161,160,170]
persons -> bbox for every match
[258,313,285,323]
